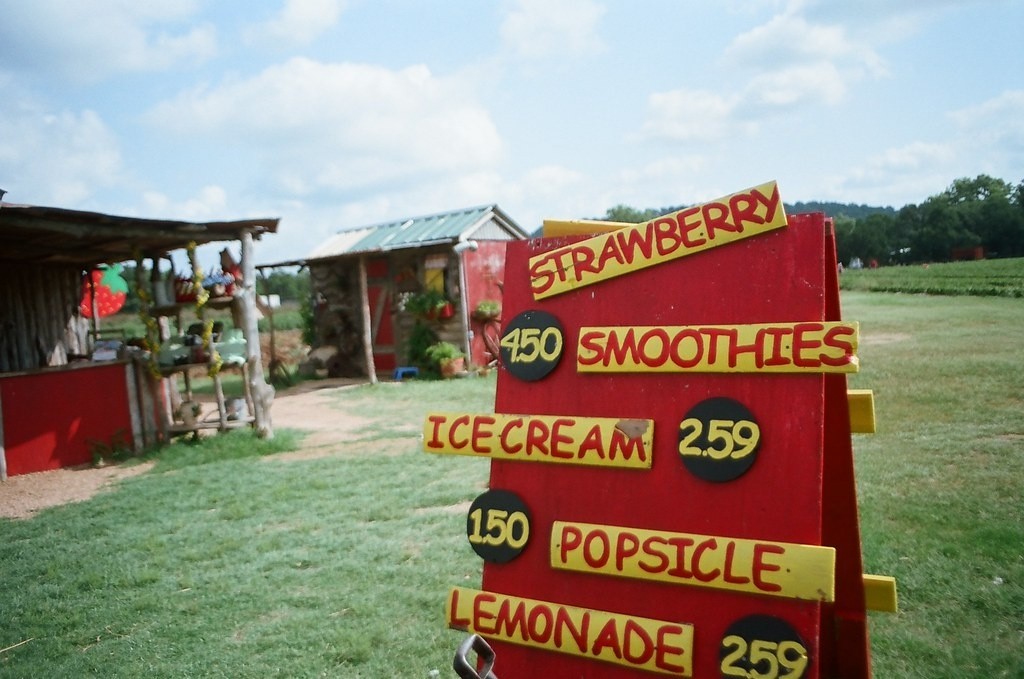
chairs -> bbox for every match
[85,328,128,361]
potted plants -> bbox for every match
[406,287,461,322]
[404,324,466,379]
[474,301,501,319]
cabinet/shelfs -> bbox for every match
[140,294,257,450]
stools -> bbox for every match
[391,367,420,381]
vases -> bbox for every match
[173,400,202,426]
